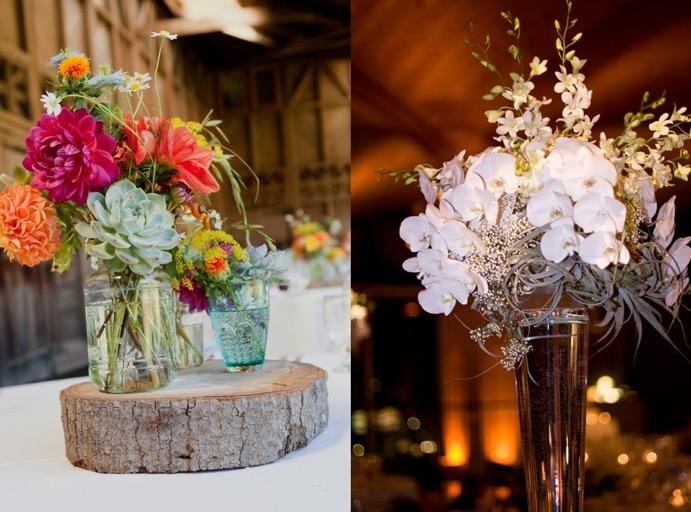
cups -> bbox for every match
[84,274,271,392]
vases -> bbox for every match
[82,267,176,395]
[513,285,589,511]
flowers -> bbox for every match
[372,0,690,385]
[0,30,351,389]
